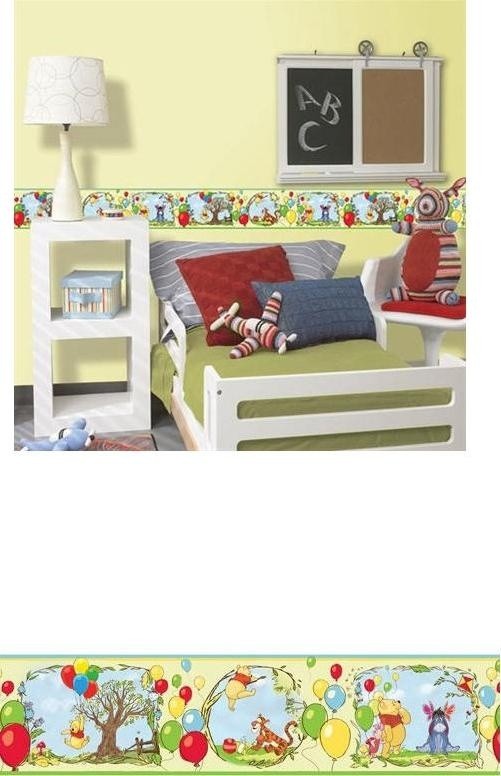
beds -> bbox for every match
[158,289,466,451]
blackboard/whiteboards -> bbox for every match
[274,55,444,184]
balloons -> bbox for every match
[0,655,499,770]
[448,195,461,222]
[15,191,49,227]
[90,189,415,228]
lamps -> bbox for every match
[24,56,110,222]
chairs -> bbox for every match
[361,238,467,366]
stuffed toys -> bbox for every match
[206,290,297,360]
[385,175,465,303]
[17,418,96,451]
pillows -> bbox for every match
[136,240,376,351]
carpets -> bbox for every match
[82,433,158,450]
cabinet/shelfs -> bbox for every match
[30,216,152,438]
